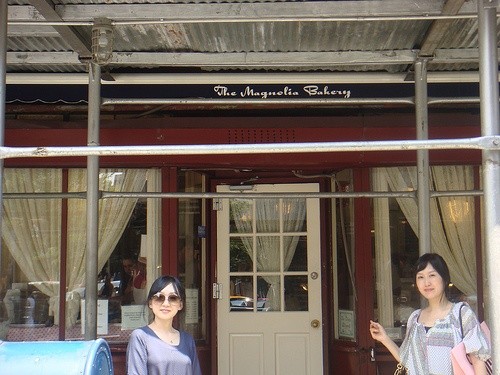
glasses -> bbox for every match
[151,293,181,304]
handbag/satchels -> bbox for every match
[449,303,491,375]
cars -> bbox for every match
[254,298,271,312]
[228,295,252,311]
